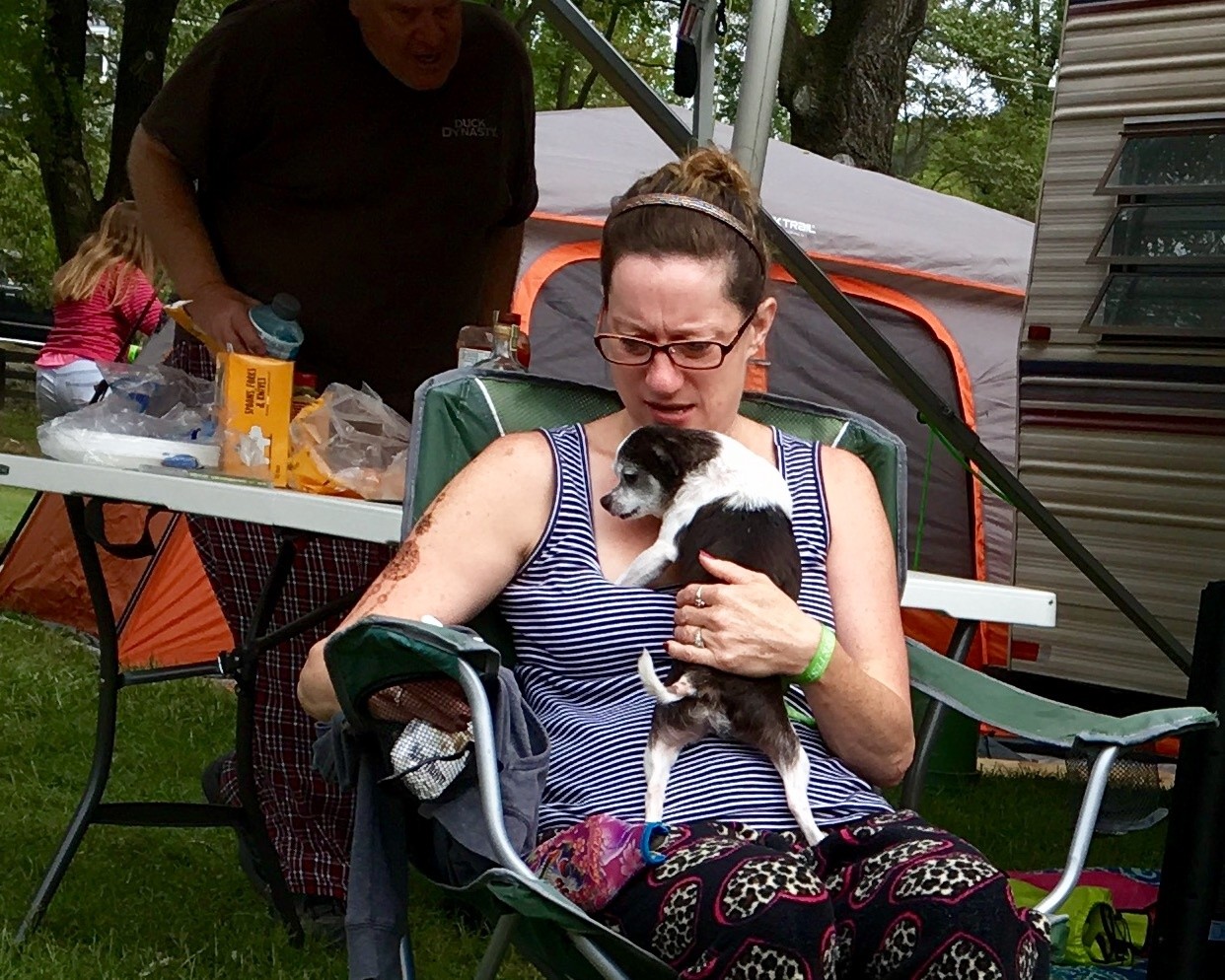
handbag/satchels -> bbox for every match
[288,381,411,505]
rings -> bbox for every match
[695,585,705,607]
[394,688,403,707]
[695,627,704,648]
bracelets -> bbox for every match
[780,623,836,687]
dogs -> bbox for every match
[598,424,828,849]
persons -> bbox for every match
[34,199,167,423]
[126,0,539,954]
[296,147,1051,980]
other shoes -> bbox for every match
[203,756,269,893]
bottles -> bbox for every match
[498,312,531,369]
[472,325,529,373]
[246,292,305,358]
[288,373,331,462]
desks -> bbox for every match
[1,453,1056,979]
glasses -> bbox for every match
[591,295,759,370]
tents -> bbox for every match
[0,105,1034,671]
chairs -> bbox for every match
[324,378,1216,980]
